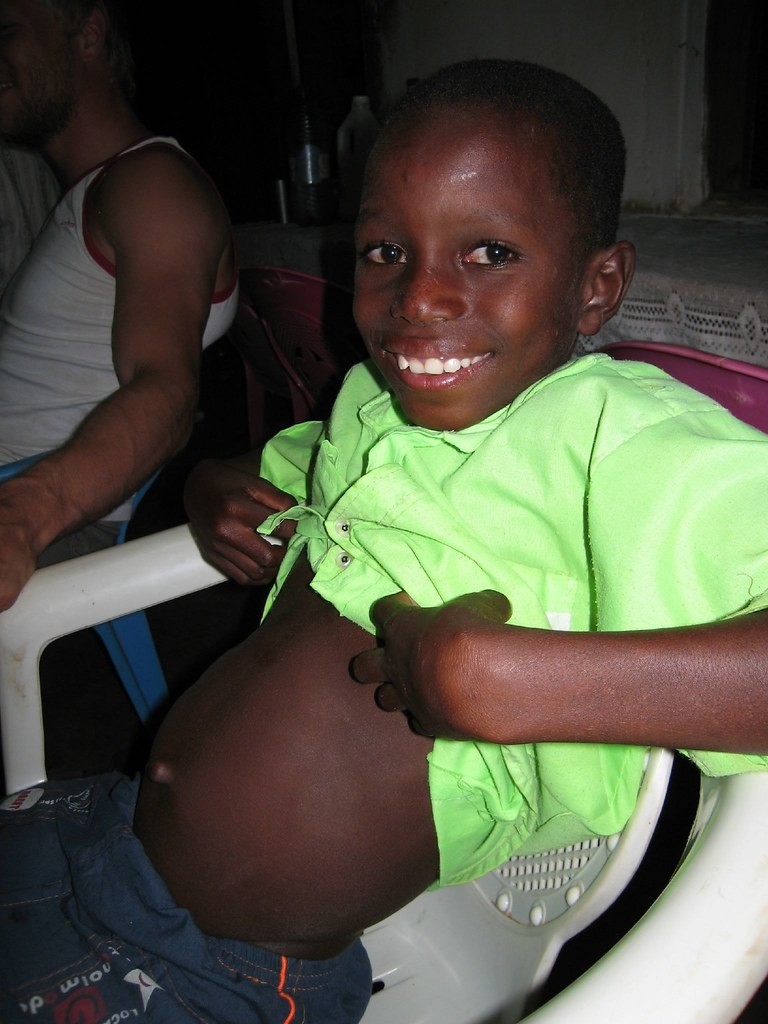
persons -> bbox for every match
[0,0,240,611]
[0,54,768,1024]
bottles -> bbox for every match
[286,85,337,227]
[336,95,380,220]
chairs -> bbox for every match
[0,266,768,1024]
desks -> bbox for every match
[229,210,768,362]
